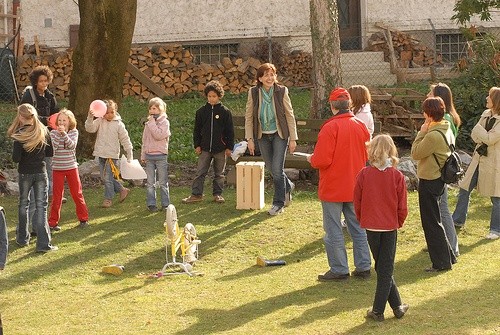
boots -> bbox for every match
[256,256,286,267]
[101,263,125,274]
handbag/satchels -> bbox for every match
[236,137,261,156]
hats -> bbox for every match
[328,87,352,102]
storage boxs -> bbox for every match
[235,161,266,210]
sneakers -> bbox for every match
[268,206,284,215]
[485,231,500,239]
[102,200,112,208]
[118,187,130,203]
[15,240,30,247]
[38,244,57,253]
[284,183,295,206]
[452,221,466,227]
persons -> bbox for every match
[140,96,171,211]
[21,65,68,202]
[339,85,375,227]
[85,98,133,208]
[184,79,236,202]
[246,62,298,215]
[353,132,411,320]
[8,103,59,251]
[411,95,456,272]
[424,82,461,256]
[308,86,373,280]
[0,171,9,335]
[452,86,500,240]
[47,108,88,230]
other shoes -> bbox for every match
[215,195,225,202]
[351,267,371,278]
[52,224,61,231]
[182,195,203,203]
[81,221,88,228]
[422,246,429,252]
[367,309,384,321]
[62,198,67,202]
[340,219,346,227]
[394,302,410,319]
[425,266,452,272]
[149,206,168,213]
[317,269,351,281]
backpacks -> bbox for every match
[429,129,463,184]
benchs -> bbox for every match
[226,116,327,186]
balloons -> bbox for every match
[89,100,107,118]
[49,112,59,130]
